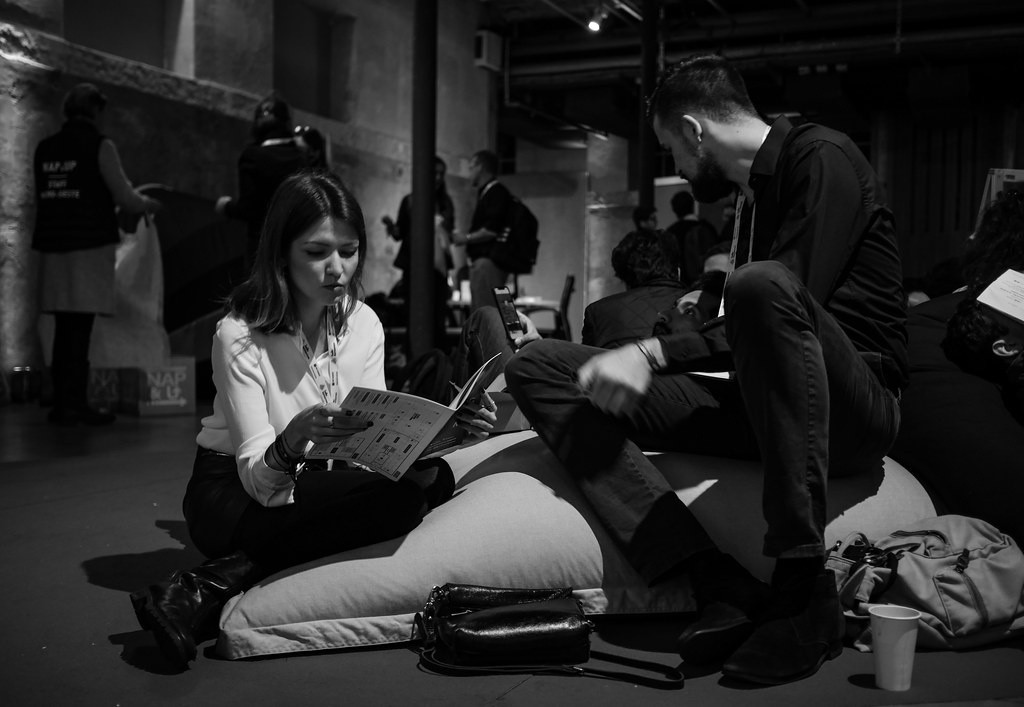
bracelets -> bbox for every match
[271,431,309,482]
[635,338,661,374]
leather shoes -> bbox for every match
[680,552,772,666]
[722,568,849,688]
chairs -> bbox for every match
[521,274,575,343]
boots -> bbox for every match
[128,548,273,669]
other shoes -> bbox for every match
[49,406,118,425]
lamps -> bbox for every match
[585,9,610,35]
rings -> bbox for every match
[329,416,334,429]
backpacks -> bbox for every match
[495,184,540,275]
[826,513,1024,653]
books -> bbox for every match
[306,351,503,482]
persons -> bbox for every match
[32,83,161,458]
[217,97,728,406]
[130,170,497,662]
[504,54,912,685]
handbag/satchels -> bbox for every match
[409,582,685,691]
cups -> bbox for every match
[868,604,921,692]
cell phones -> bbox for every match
[491,286,525,340]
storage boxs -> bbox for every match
[118,357,197,420]
